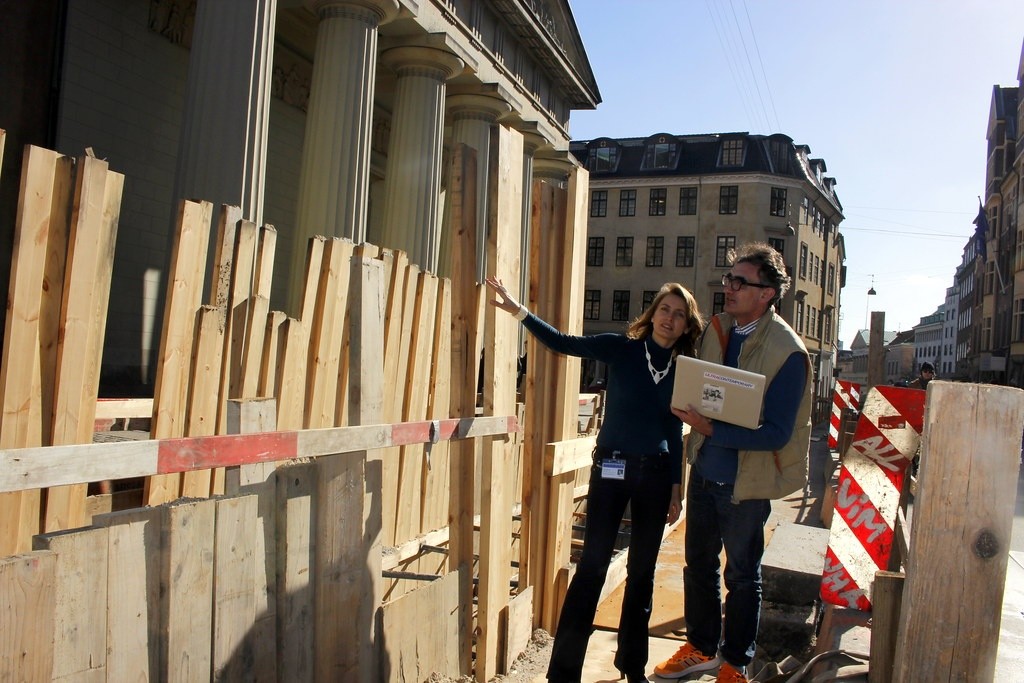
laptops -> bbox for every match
[671,355,767,430]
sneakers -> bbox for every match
[714,661,749,683]
[654,642,720,678]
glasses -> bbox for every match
[720,274,764,291]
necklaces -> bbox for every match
[644,341,673,384]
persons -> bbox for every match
[907,361,936,390]
[887,376,908,388]
[516,339,528,390]
[484,275,703,682]
[650,242,813,683]
